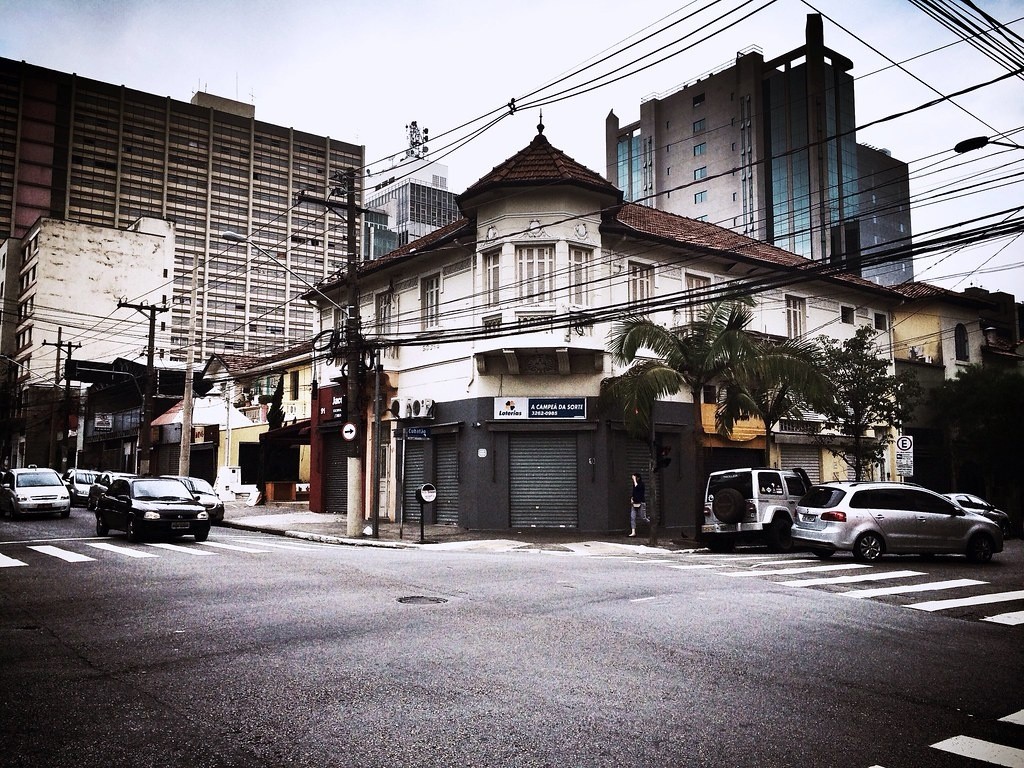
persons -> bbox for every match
[628,472,651,537]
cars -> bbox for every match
[87,472,139,512]
[94,477,211,542]
[63,467,102,507]
[160,474,224,527]
[0,464,71,521]
[940,493,1012,536]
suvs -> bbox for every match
[790,480,1004,569]
[701,468,815,554]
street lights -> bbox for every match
[222,230,362,539]
[0,354,70,475]
